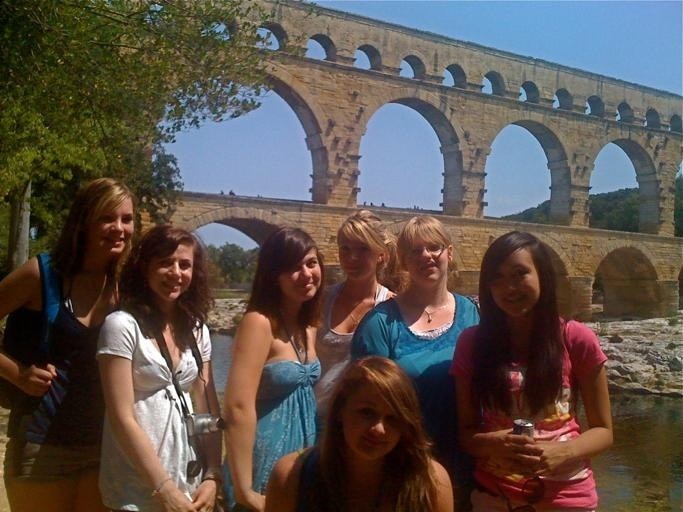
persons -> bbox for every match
[223,225,326,512]
[314,207,397,439]
[352,213,478,444]
[95,224,222,512]
[448,230,613,512]
[266,356,454,512]
[0,177,136,512]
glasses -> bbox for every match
[406,244,444,256]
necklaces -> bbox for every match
[417,302,450,323]
[349,305,367,327]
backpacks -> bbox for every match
[0,252,62,410]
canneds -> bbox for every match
[513,419,534,439]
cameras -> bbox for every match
[187,413,224,436]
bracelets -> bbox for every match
[202,467,223,481]
[151,478,174,497]
